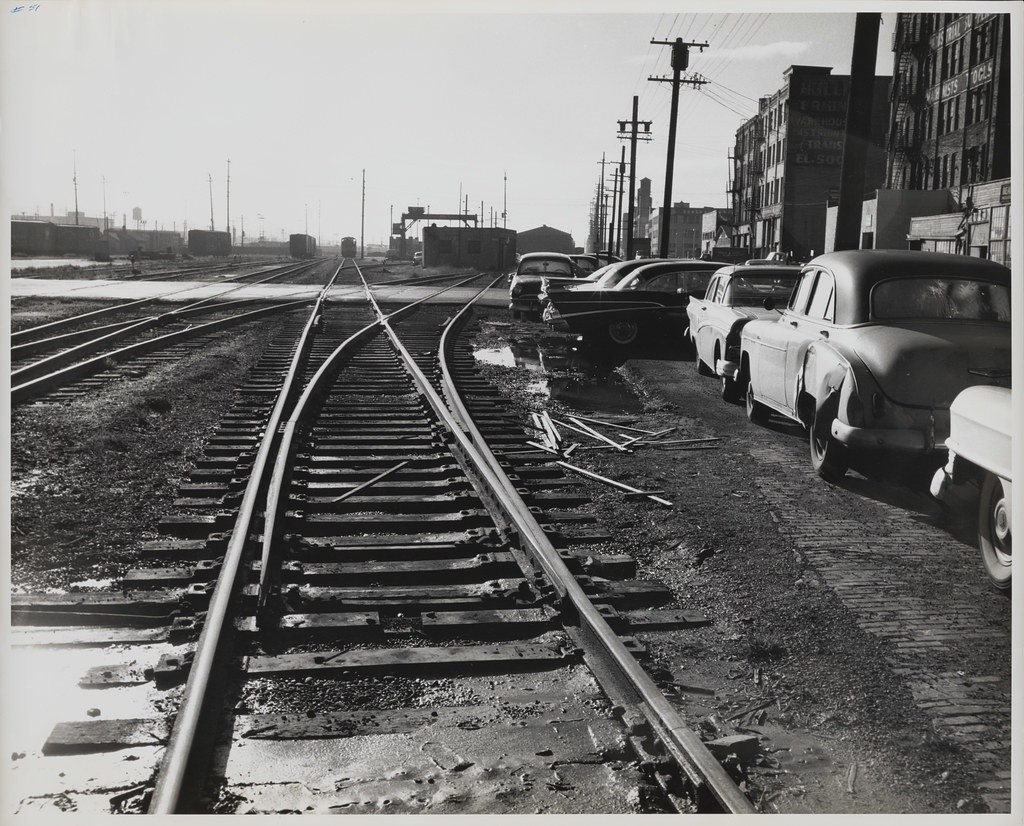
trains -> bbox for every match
[340,236,356,258]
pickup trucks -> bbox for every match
[684,259,822,403]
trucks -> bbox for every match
[745,251,803,267]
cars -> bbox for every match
[589,252,626,265]
[567,255,607,280]
[538,257,704,320]
[508,250,580,319]
[543,260,778,348]
[739,248,1012,481]
[944,382,1012,591]
